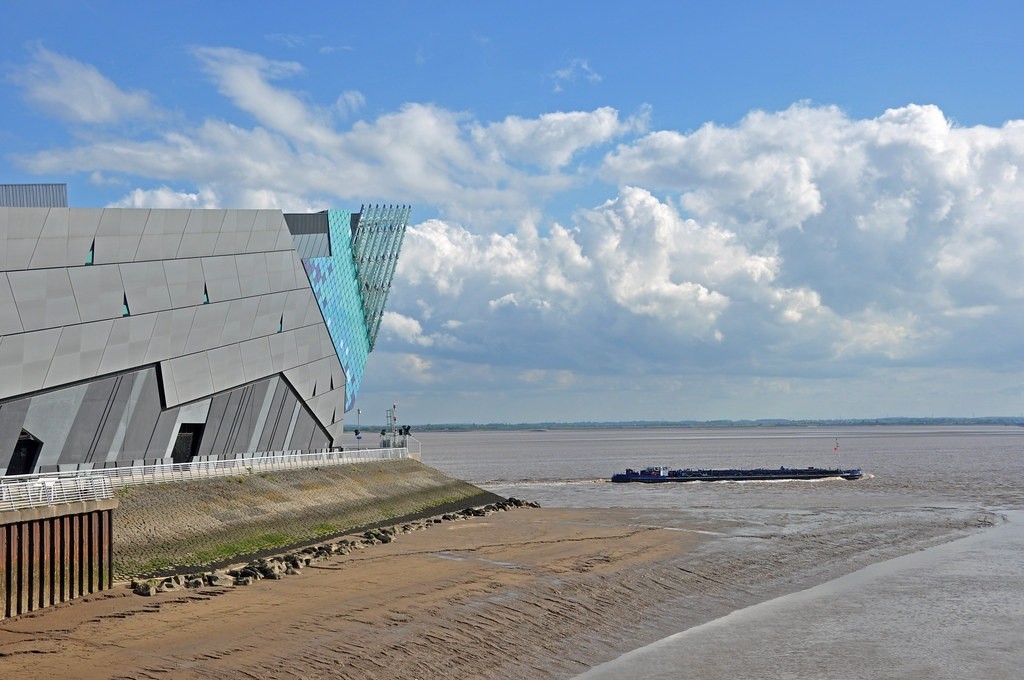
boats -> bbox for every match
[609,464,863,483]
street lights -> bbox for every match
[357,408,361,450]
